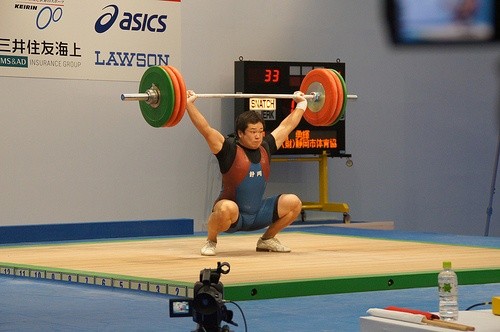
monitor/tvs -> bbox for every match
[384,0,500,43]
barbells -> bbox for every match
[121,68,358,128]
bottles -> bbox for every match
[436,258,461,323]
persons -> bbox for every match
[185,90,307,256]
